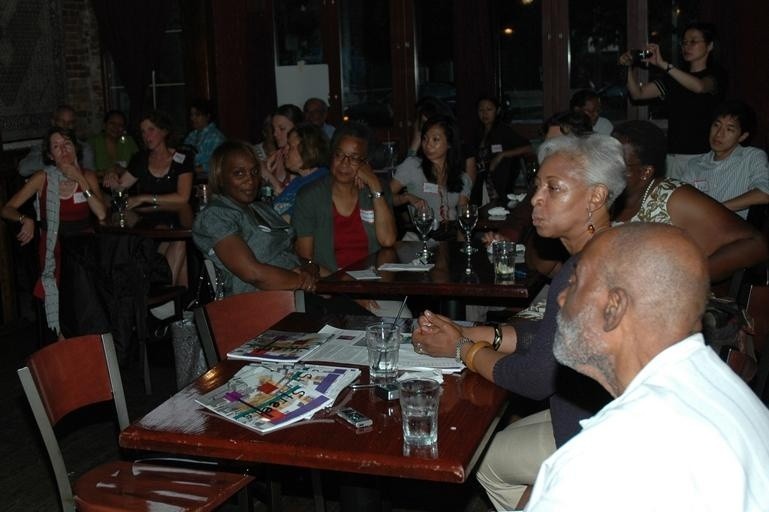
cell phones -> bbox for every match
[336,407,373,429]
[374,382,403,400]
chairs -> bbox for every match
[16,330,256,509]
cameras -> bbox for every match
[630,48,653,69]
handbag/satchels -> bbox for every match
[171,310,208,391]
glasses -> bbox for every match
[333,150,362,166]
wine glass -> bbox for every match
[111,193,131,228]
[459,255,481,286]
[413,212,436,258]
[457,203,480,254]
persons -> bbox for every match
[411,131,630,511]
[517,221,768,512]
[0,27,768,433]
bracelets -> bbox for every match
[455,338,473,363]
[485,324,503,351]
[465,341,494,374]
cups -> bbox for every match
[398,376,441,446]
[369,378,388,402]
[494,275,517,286]
[365,322,402,379]
[403,443,441,460]
[493,240,517,278]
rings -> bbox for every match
[414,347,425,353]
[416,341,423,347]
